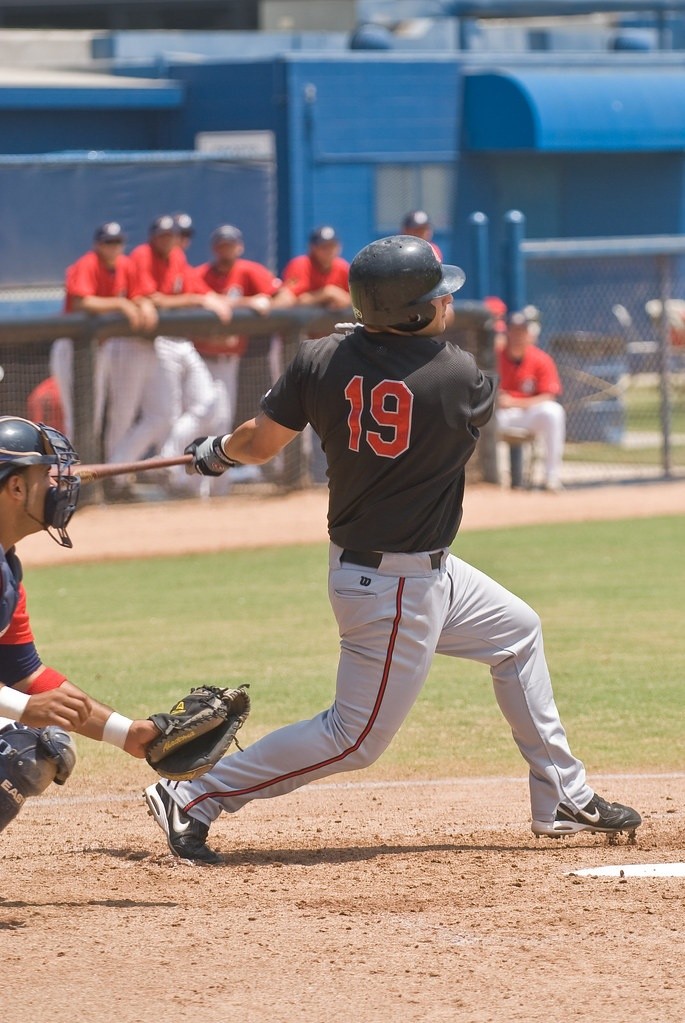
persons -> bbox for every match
[48,213,353,499]
[468,294,567,487]
[401,211,443,261]
[145,235,644,857]
[0,415,250,837]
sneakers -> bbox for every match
[531,793,641,839]
[142,782,222,864]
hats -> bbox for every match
[212,225,240,240]
[311,227,337,244]
[402,211,430,228]
[95,221,125,242]
[150,213,197,236]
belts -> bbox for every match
[339,548,443,569]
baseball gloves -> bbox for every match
[144,683,251,783]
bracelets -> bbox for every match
[0,686,31,722]
[102,711,135,749]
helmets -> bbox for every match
[0,415,47,479]
[349,235,466,334]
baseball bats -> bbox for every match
[53,453,198,484]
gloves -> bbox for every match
[184,434,239,477]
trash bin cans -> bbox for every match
[554,329,625,443]
